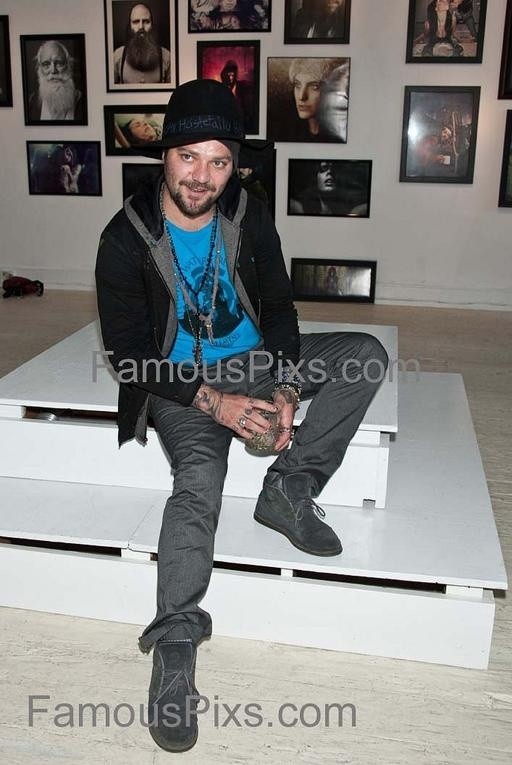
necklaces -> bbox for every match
[159,183,221,367]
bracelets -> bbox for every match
[270,375,303,412]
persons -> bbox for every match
[218,60,253,130]
[58,147,83,194]
[289,59,347,144]
[29,40,82,120]
[420,0,478,56]
[111,116,161,151]
[115,4,171,84]
[198,0,260,29]
[94,80,389,754]
[414,126,454,176]
[291,0,344,38]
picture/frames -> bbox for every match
[266,56,352,144]
[234,144,275,228]
[399,86,481,185]
[188,0,272,32]
[497,109,512,209]
[19,32,88,125]
[25,139,103,196]
[103,0,179,92]
[1,14,13,106]
[498,0,512,102]
[103,105,166,157]
[284,1,352,45]
[289,258,377,304]
[405,1,487,64]
[196,40,260,135]
[123,162,162,201]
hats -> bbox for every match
[133,79,245,152]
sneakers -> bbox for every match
[254,473,342,556]
[149,641,200,752]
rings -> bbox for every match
[240,416,248,428]
[279,426,292,433]
